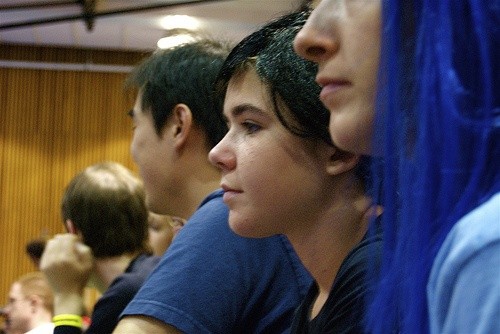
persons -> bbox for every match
[37,161,166,334]
[108,35,313,334]
[1,271,53,334]
[291,1,482,334]
[206,6,390,334]
[147,210,186,258]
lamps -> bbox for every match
[157,28,197,50]
